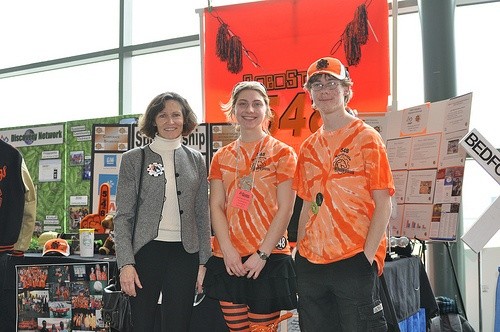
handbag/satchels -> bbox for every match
[101,283,133,332]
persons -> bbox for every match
[202,81,297,332]
[292,57,394,332]
[114,93,209,332]
[0,137,37,332]
[19,263,108,332]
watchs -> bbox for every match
[256,250,270,261]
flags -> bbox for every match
[204,0,389,157]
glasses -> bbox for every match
[311,81,343,91]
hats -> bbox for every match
[39,231,58,250]
[306,57,348,84]
[43,238,70,256]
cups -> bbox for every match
[79,229,95,257]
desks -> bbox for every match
[154,254,440,332]
[0,252,122,332]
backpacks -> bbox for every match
[429,313,475,332]
